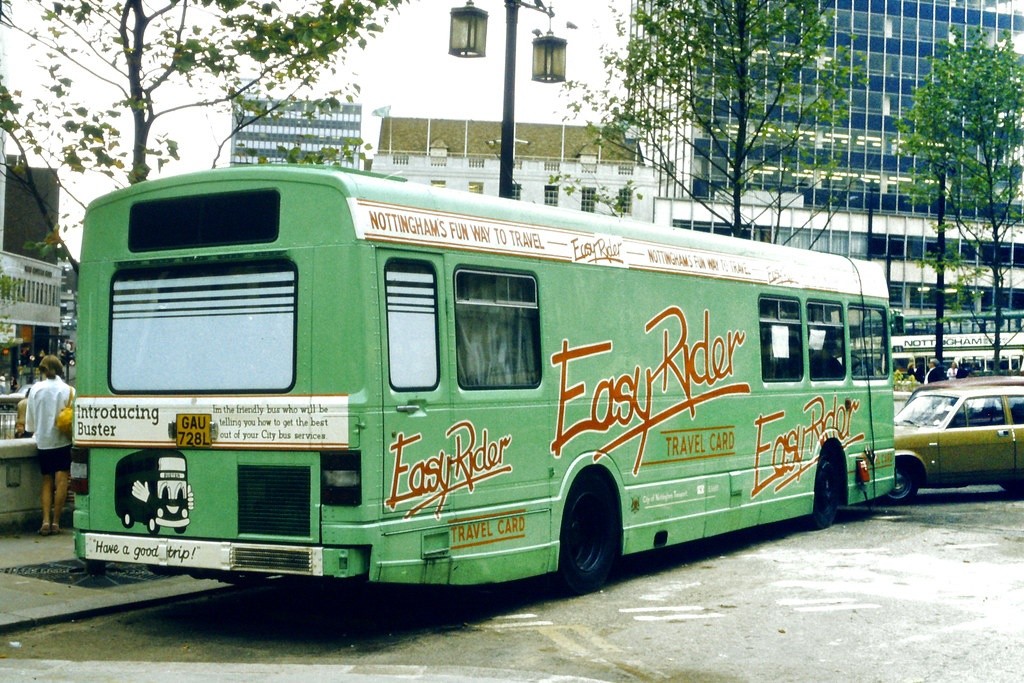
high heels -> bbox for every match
[39,523,51,535]
[52,523,60,534]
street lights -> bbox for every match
[450,0,575,199]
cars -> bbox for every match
[876,376,1024,507]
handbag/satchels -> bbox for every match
[57,388,74,441]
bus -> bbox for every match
[73,166,904,595]
[853,310,1024,377]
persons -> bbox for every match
[924,358,943,384]
[10,375,17,393]
[893,362,903,381]
[18,388,31,425]
[25,355,75,536]
[15,423,24,438]
[946,363,980,380]
[907,362,924,383]
